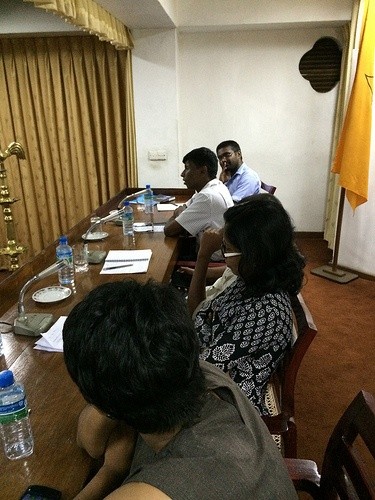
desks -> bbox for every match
[0,187,204,500]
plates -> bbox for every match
[81,232,109,240]
[32,286,71,303]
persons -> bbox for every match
[62,278,299,500]
[196,200,308,417]
[163,140,261,319]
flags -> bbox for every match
[337,0,375,217]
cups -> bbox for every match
[90,217,102,237]
[72,244,89,273]
[136,194,144,211]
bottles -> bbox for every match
[144,185,154,213]
[59,283,77,295]
[122,201,134,235]
[56,236,75,284]
[0,370,34,460]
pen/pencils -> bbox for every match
[104,264,133,271]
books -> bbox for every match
[100,249,152,275]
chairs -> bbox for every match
[177,259,224,268]
[261,291,318,459]
[286,389,375,500]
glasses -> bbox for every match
[220,240,242,258]
[217,151,234,160]
